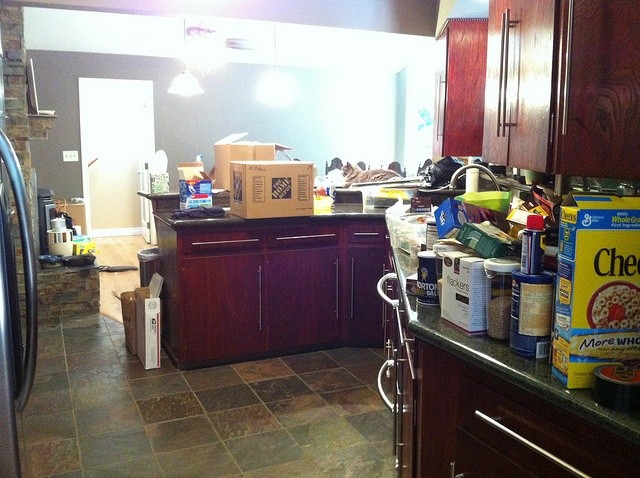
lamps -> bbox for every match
[167,73,204,98]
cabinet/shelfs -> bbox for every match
[433,19,488,157]
[482,0,639,180]
[269,220,390,357]
[419,344,640,478]
[389,251,418,478]
[156,220,268,370]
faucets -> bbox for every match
[448,164,502,192]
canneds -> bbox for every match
[415,249,438,309]
[510,266,557,360]
[521,228,547,273]
[426,217,438,251]
[484,255,521,342]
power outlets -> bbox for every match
[62,150,79,162]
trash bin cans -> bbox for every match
[138,246,159,288]
